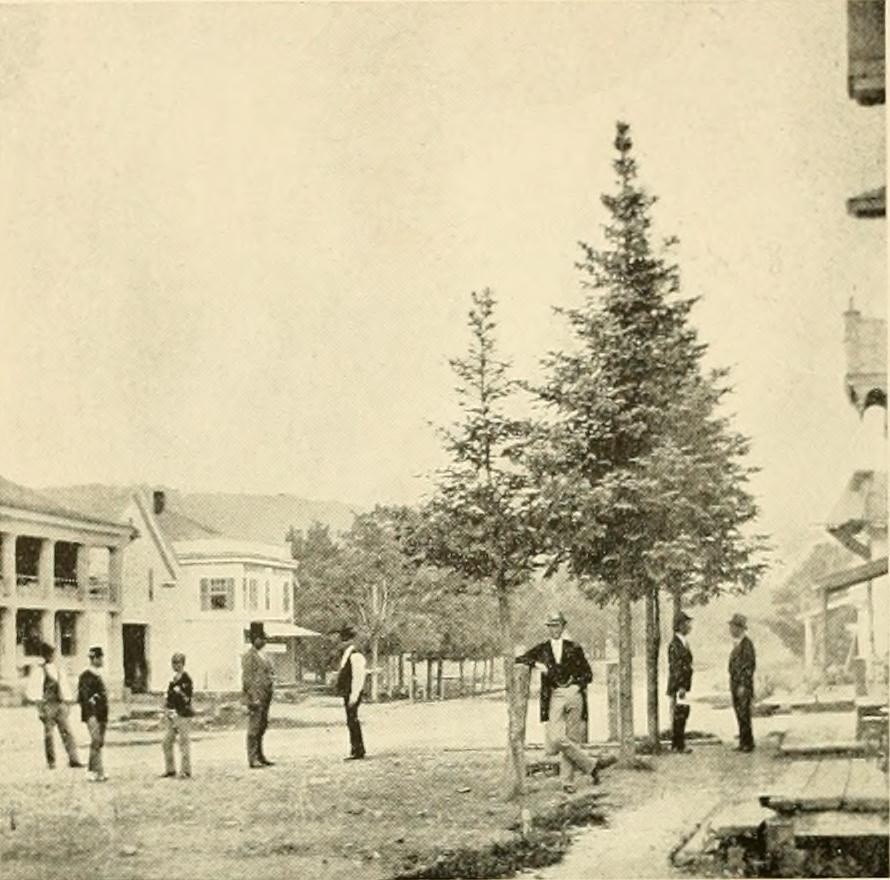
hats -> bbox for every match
[40,643,54,657]
[248,622,264,637]
[543,611,569,625]
[328,621,358,640]
[727,613,750,631]
[672,607,692,630]
[88,647,103,658]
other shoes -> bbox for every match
[592,762,602,785]
[86,772,109,781]
[249,759,273,768]
[564,785,577,792]
[671,745,693,754]
[161,771,192,779]
[344,753,365,761]
[733,743,755,752]
[48,760,87,768]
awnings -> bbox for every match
[245,621,323,641]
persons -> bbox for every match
[156,650,195,780]
[238,618,278,768]
[28,639,89,768]
[77,645,110,781]
[516,608,601,786]
[665,610,699,755]
[725,610,761,753]
[333,626,369,760]
